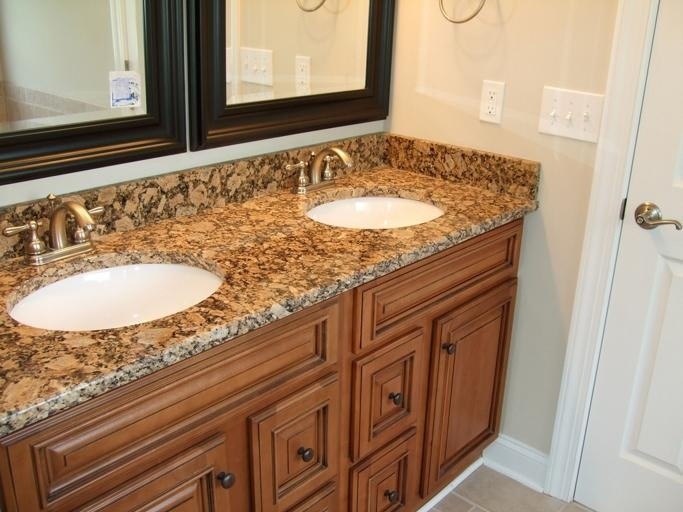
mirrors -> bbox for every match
[188,0,398,154]
[0,1,188,187]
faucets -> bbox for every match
[48,200,98,250]
[309,146,356,184]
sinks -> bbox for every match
[302,185,449,230]
[5,248,227,333]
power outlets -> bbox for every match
[293,55,309,92]
[479,79,505,125]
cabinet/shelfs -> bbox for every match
[0,217,524,512]
